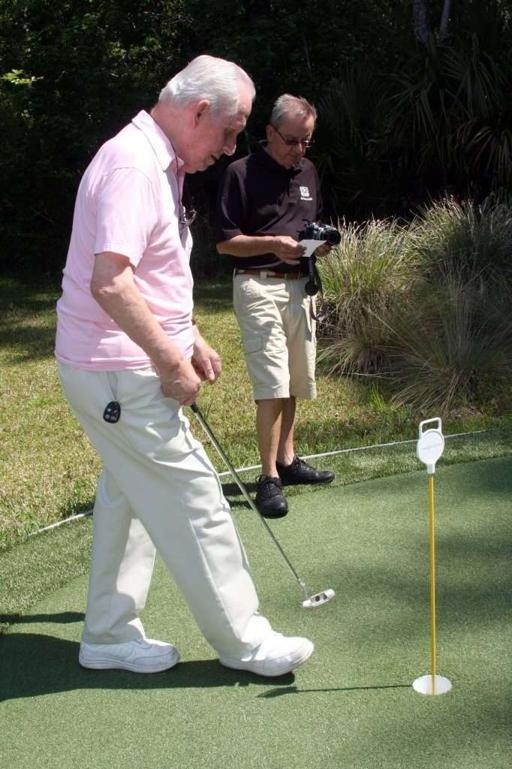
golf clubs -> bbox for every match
[191,400,336,609]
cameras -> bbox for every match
[298,222,341,246]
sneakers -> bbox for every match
[276,456,336,485]
[217,627,316,677]
[254,473,288,518]
[78,637,181,675]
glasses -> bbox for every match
[179,205,198,231]
[271,123,315,149]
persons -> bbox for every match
[214,89,334,518]
[55,54,315,675]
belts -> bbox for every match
[235,268,309,280]
